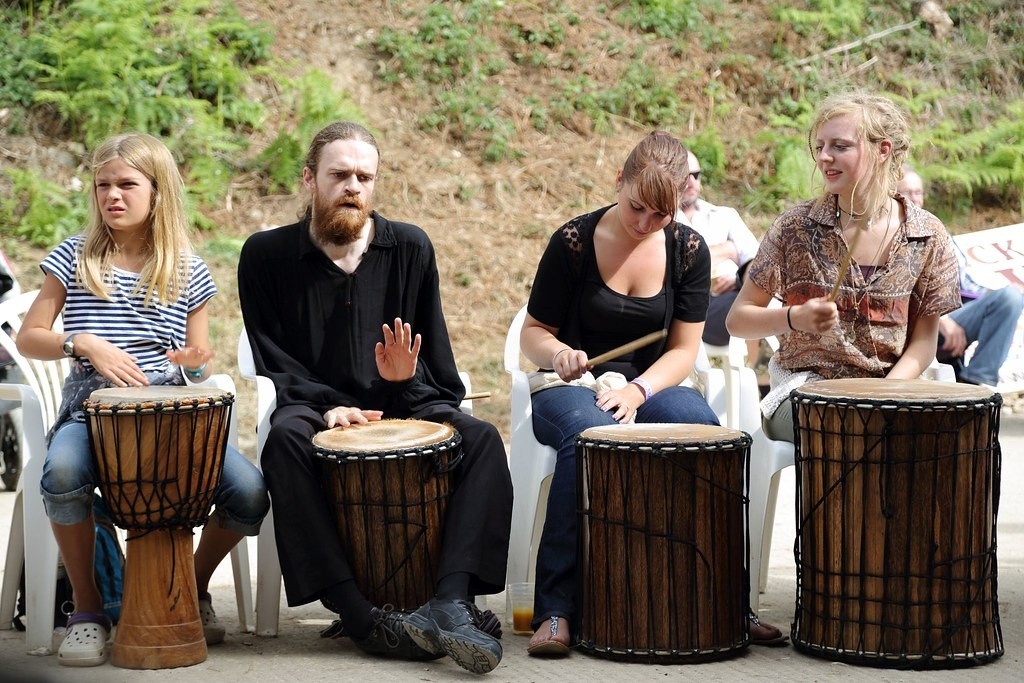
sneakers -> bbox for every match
[403,597,503,674]
[353,604,447,661]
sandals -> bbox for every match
[527,616,570,654]
[748,608,789,644]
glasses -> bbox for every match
[688,171,701,180]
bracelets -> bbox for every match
[184,365,207,377]
[787,305,796,331]
[631,378,651,401]
[552,349,571,361]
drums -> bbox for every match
[790,378,1005,670]
[310,418,463,614]
[83,386,235,670]
[574,423,752,666]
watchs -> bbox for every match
[62,334,82,360]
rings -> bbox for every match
[623,404,629,409]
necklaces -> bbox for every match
[837,198,892,310]
[839,207,889,228]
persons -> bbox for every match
[722,91,962,446]
[520,132,789,656]
[670,149,759,347]
[16,131,270,666]
[238,121,514,673]
[893,164,1024,387]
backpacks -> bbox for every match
[19,493,125,627]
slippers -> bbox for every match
[200,592,225,644]
[58,614,112,666]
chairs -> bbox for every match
[728,286,957,617]
[239,322,474,638]
[0,285,257,655]
[502,300,726,625]
